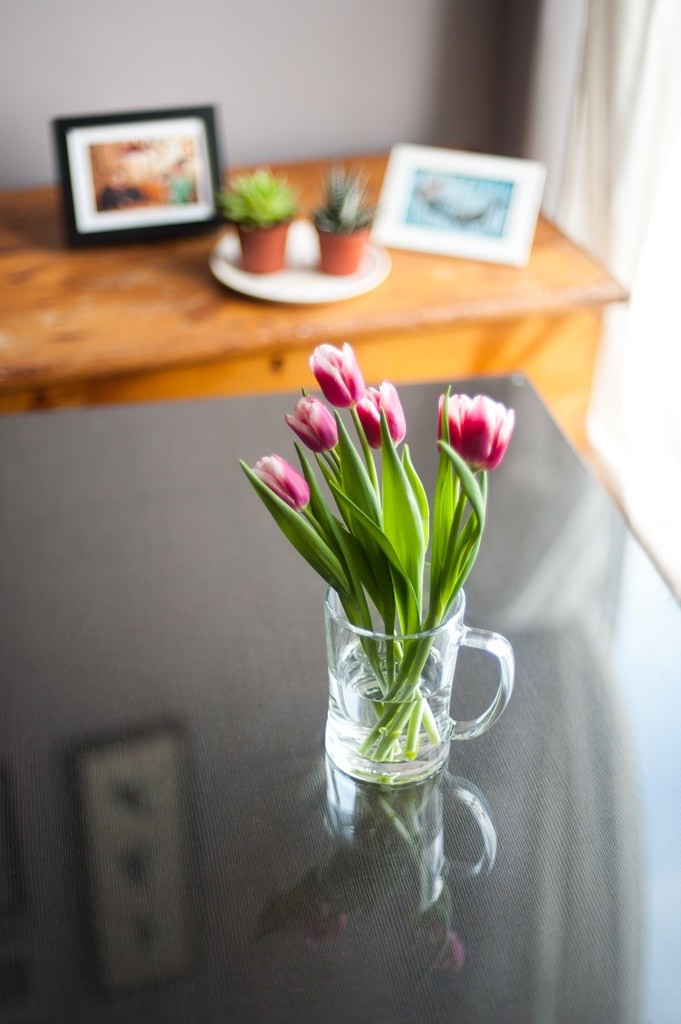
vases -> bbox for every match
[322,587,515,787]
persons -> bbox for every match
[100,174,143,209]
[165,161,193,203]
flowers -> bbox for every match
[252,776,498,991]
[242,339,513,601]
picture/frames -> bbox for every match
[49,103,229,245]
[368,143,545,267]
[60,715,208,993]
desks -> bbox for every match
[0,370,680,1024]
[0,147,632,445]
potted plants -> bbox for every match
[309,168,377,275]
[218,165,299,273]
